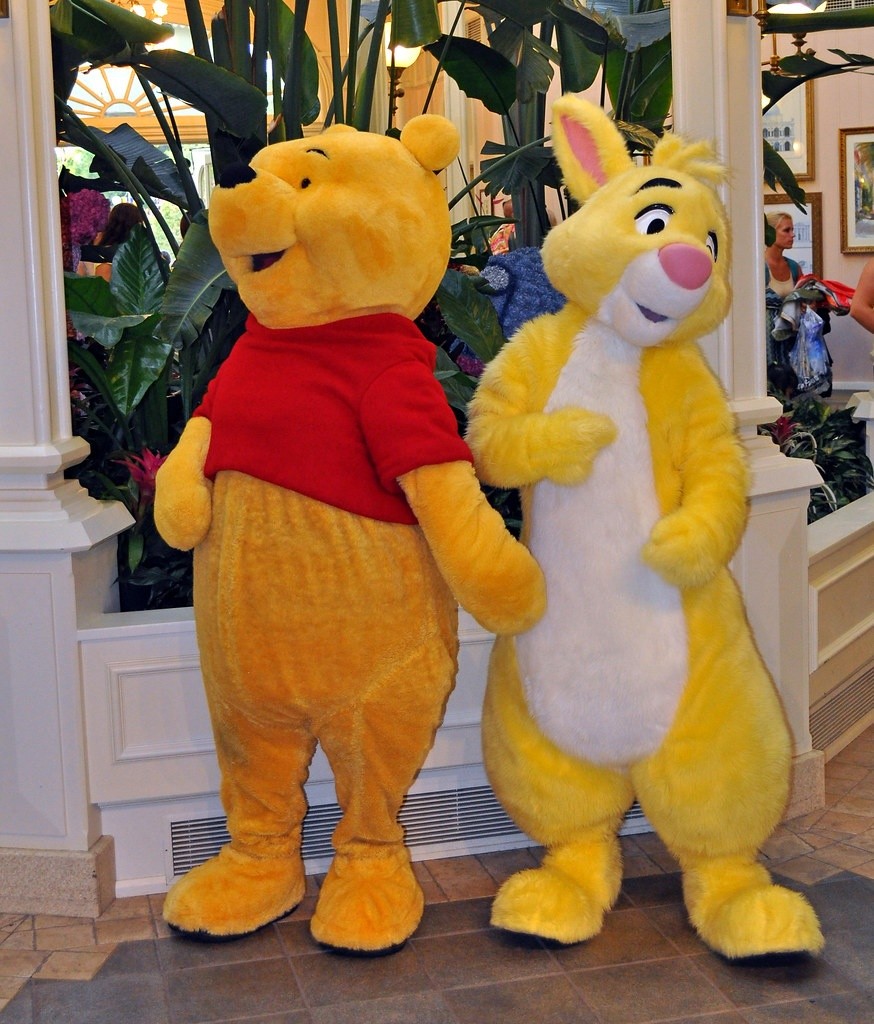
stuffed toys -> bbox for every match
[154,113,547,955]
[466,93,827,969]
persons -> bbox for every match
[764,210,805,400]
[851,258,874,334]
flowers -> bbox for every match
[102,448,173,526]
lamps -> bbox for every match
[753,0,828,79]
[382,22,422,114]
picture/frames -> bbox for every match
[838,126,874,254]
[726,0,753,17]
[762,75,817,184]
[764,192,824,282]
